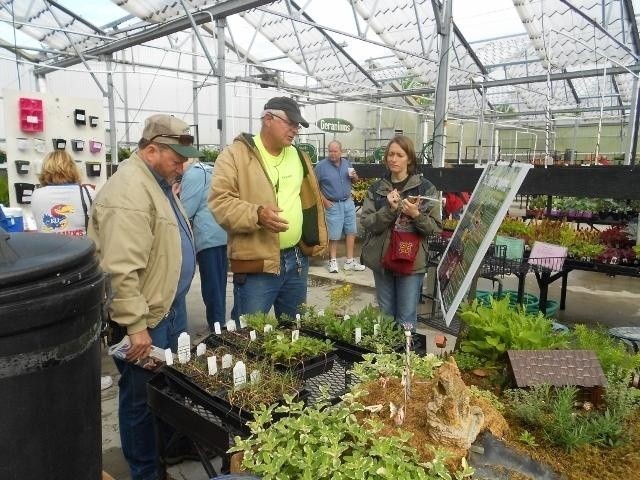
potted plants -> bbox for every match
[281,313,427,363]
[208,320,337,380]
[161,344,312,433]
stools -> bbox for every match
[608,326,640,388]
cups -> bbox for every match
[347,167,355,177]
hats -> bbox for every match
[142,114,206,158]
[264,96,309,128]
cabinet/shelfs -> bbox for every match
[147,304,425,478]
[429,232,574,320]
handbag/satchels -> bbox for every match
[382,229,421,275]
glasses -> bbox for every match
[261,112,303,132]
[146,134,195,146]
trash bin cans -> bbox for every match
[0,229,102,480]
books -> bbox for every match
[392,195,437,234]
[108,333,178,376]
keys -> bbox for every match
[294,247,304,277]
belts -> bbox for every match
[330,198,349,202]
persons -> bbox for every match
[311,139,365,273]
[206,97,329,332]
[88,113,206,479]
[171,155,228,333]
[31,150,97,237]
[360,135,444,336]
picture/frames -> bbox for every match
[437,160,535,326]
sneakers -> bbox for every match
[134,466,171,479]
[99,375,113,390]
[344,260,366,271]
[328,260,338,273]
[162,445,217,464]
